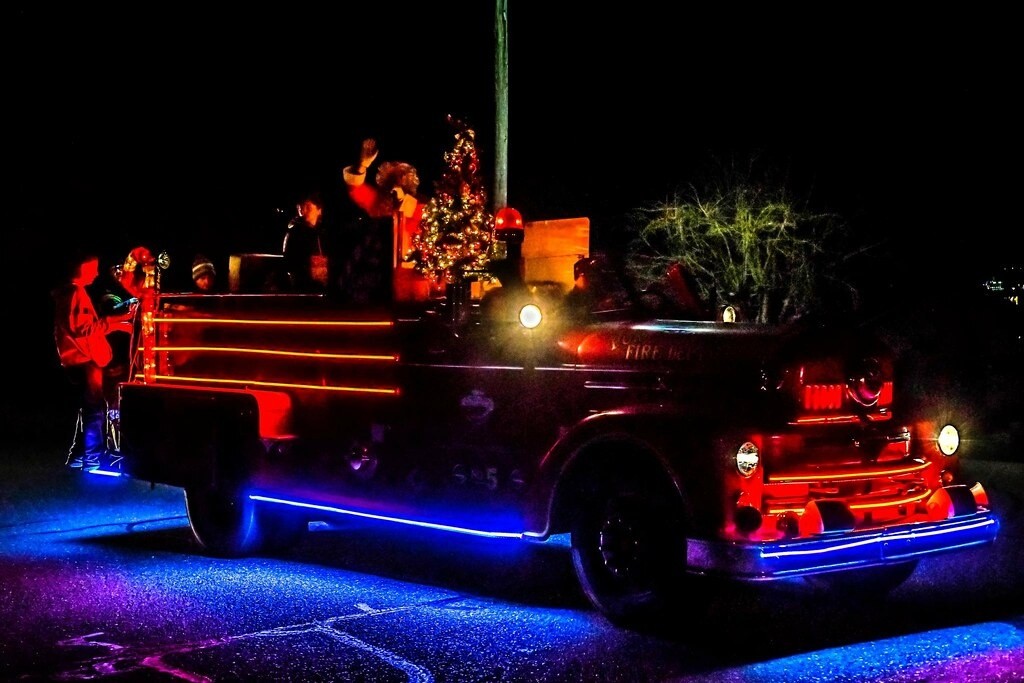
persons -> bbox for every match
[52,252,134,470]
[343,138,439,303]
[282,199,337,294]
[192,257,215,291]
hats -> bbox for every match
[191,253,216,280]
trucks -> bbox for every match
[117,211,1001,634]
